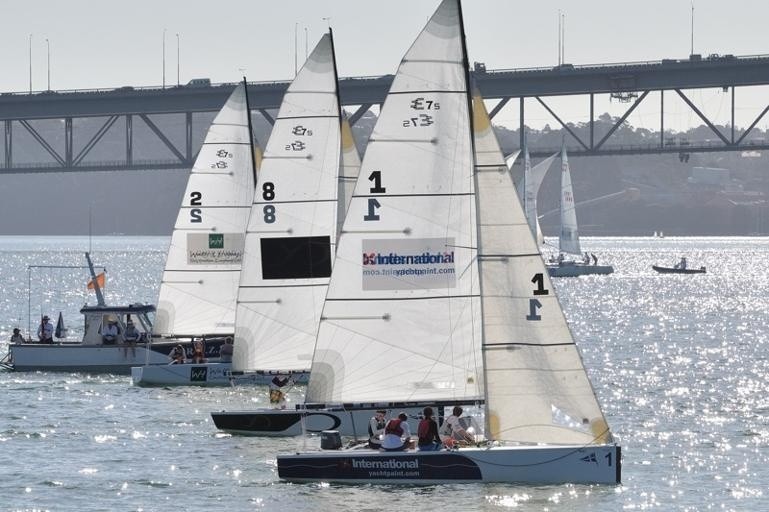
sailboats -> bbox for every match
[516,134,614,277]
[275,0,624,486]
[210,26,445,439]
[131,1,312,390]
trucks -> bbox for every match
[469,61,487,74]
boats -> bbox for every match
[0,251,234,377]
[652,264,708,274]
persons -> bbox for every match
[37,316,53,344]
[168,334,212,364]
[224,376,235,389]
[417,407,474,451]
[368,410,411,452]
[219,337,232,363]
[102,313,118,345]
[680,257,686,269]
[549,252,598,265]
[11,328,24,343]
[122,321,141,359]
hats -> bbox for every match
[376,410,386,414]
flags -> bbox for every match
[87,272,106,290]
